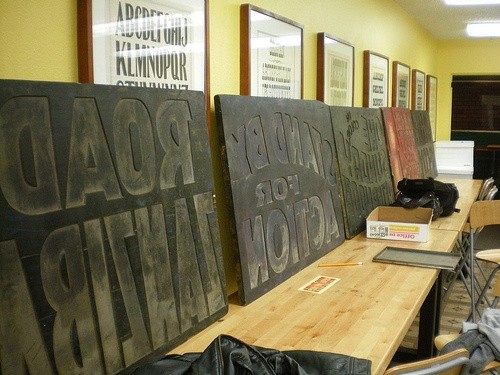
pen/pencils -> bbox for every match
[318,262,362,266]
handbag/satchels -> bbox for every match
[397,177,459,217]
[397,191,443,222]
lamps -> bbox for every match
[466,21,500,37]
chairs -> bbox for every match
[376,177,500,375]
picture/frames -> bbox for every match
[78,0,212,138]
[239,3,305,100]
[412,69,425,111]
[392,61,410,109]
[427,74,437,143]
[317,32,354,109]
[364,50,389,110]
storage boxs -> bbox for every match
[364,205,433,241]
